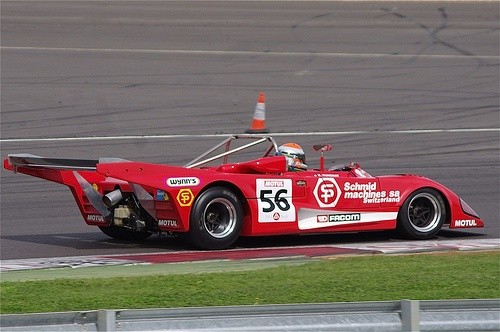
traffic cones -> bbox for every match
[245,91,272,133]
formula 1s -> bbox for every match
[5,130,487,251]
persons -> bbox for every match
[278,143,309,171]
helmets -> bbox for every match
[277,142,307,171]
